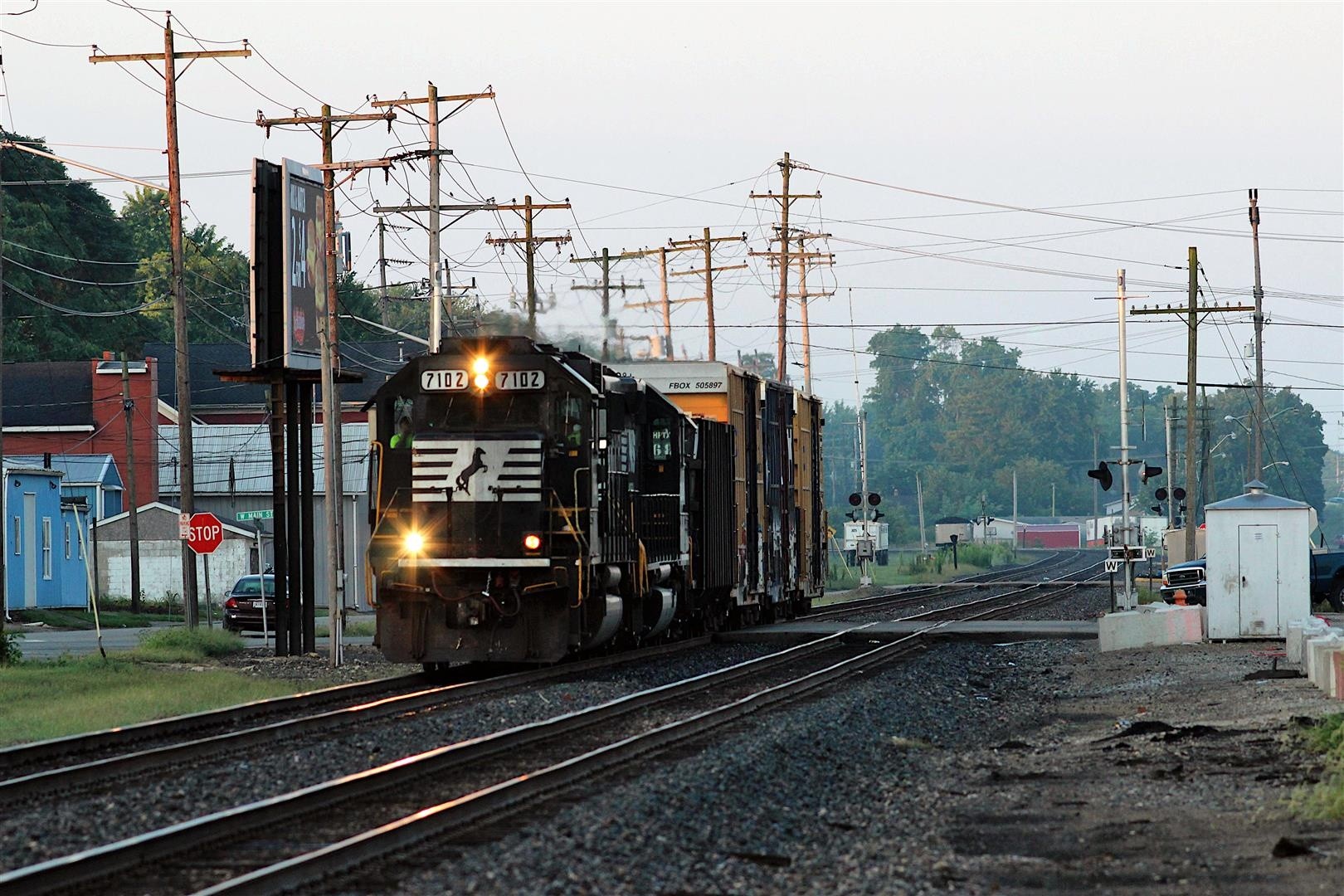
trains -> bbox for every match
[360,334,827,669]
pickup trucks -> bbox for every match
[1159,535,1344,613]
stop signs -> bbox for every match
[186,513,223,555]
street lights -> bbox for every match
[1223,405,1299,488]
[1211,432,1237,504]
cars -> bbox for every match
[221,574,303,635]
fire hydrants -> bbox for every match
[1172,589,1189,606]
